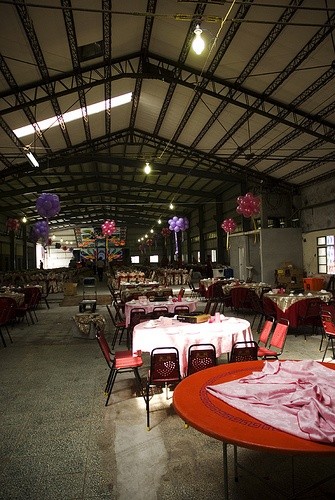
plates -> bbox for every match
[265,293,276,295]
[320,292,330,294]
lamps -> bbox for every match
[279,217,285,225]
[25,147,39,169]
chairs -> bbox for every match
[0,265,88,347]
[91,262,335,430]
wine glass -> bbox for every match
[289,289,295,296]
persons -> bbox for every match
[40,257,110,282]
[176,256,214,278]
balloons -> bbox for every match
[101,220,116,236]
[236,193,261,218]
[6,193,73,252]
[222,219,238,232]
[139,217,189,251]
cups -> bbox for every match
[139,296,146,303]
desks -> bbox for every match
[231,282,271,319]
[263,289,331,341]
[125,297,198,346]
[0,293,26,325]
[131,316,254,390]
[199,278,238,299]
[173,361,335,500]
[121,287,174,301]
[119,280,160,289]
[0,285,41,322]
